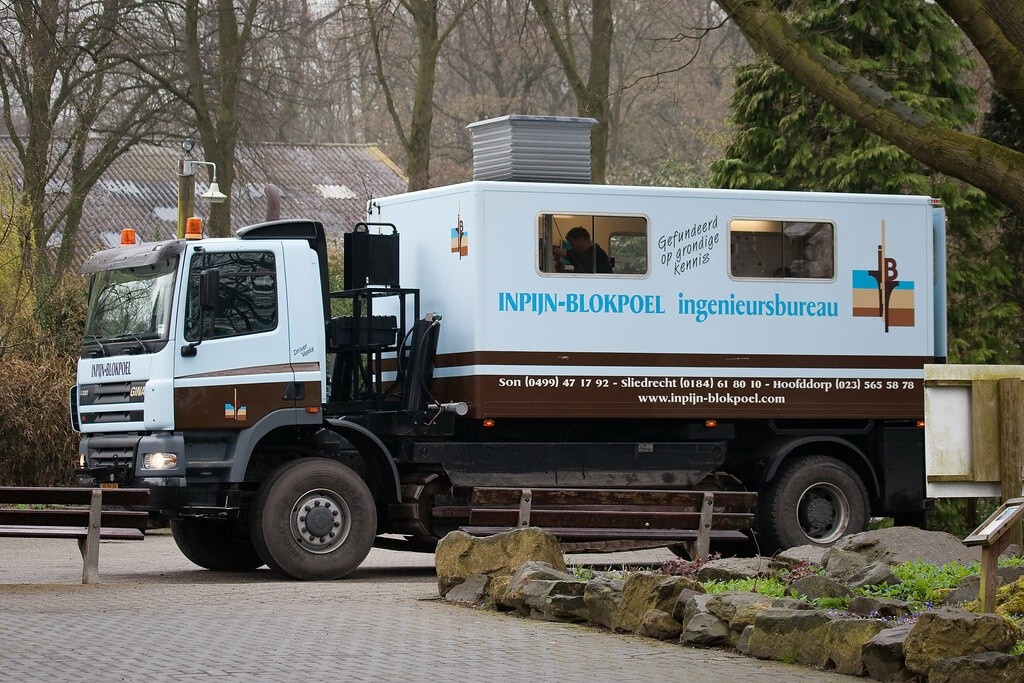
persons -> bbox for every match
[539,227,613,273]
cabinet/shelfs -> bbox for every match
[327,289,421,413]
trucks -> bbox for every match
[67,114,947,579]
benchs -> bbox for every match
[458,486,760,570]
[0,486,150,585]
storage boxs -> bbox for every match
[332,315,397,345]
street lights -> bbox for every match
[177,157,227,240]
